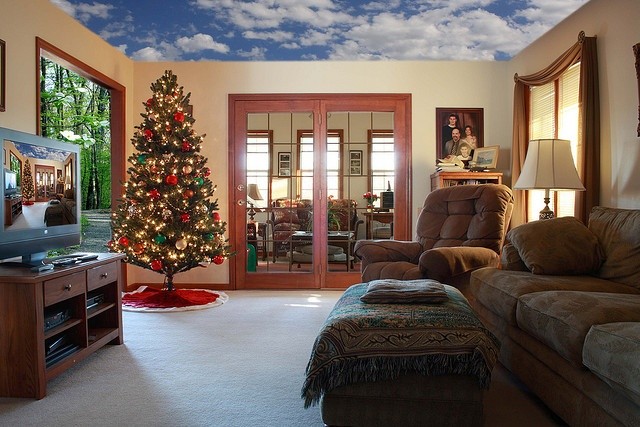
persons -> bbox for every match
[460,125,477,150]
[443,114,458,145]
[445,128,466,154]
[7,174,15,189]
[457,142,472,165]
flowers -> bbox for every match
[362,191,378,204]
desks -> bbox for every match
[361,212,394,240]
[248,222,268,260]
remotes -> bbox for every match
[78,254,98,262]
[32,263,54,274]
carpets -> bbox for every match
[122,285,230,313]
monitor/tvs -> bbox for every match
[1,127,81,270]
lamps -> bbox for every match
[247,184,265,219]
[513,139,587,220]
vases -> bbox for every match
[367,204,375,209]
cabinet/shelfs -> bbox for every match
[86,253,126,357]
[0,252,86,400]
[429,171,504,193]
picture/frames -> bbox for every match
[349,150,363,176]
[471,145,501,169]
[278,151,293,178]
[436,107,485,166]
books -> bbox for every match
[437,162,470,172]
[444,180,479,186]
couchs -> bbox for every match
[355,183,516,285]
[301,279,502,427]
[469,206,640,427]
[265,198,364,271]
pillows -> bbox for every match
[360,278,449,305]
[505,216,609,276]
